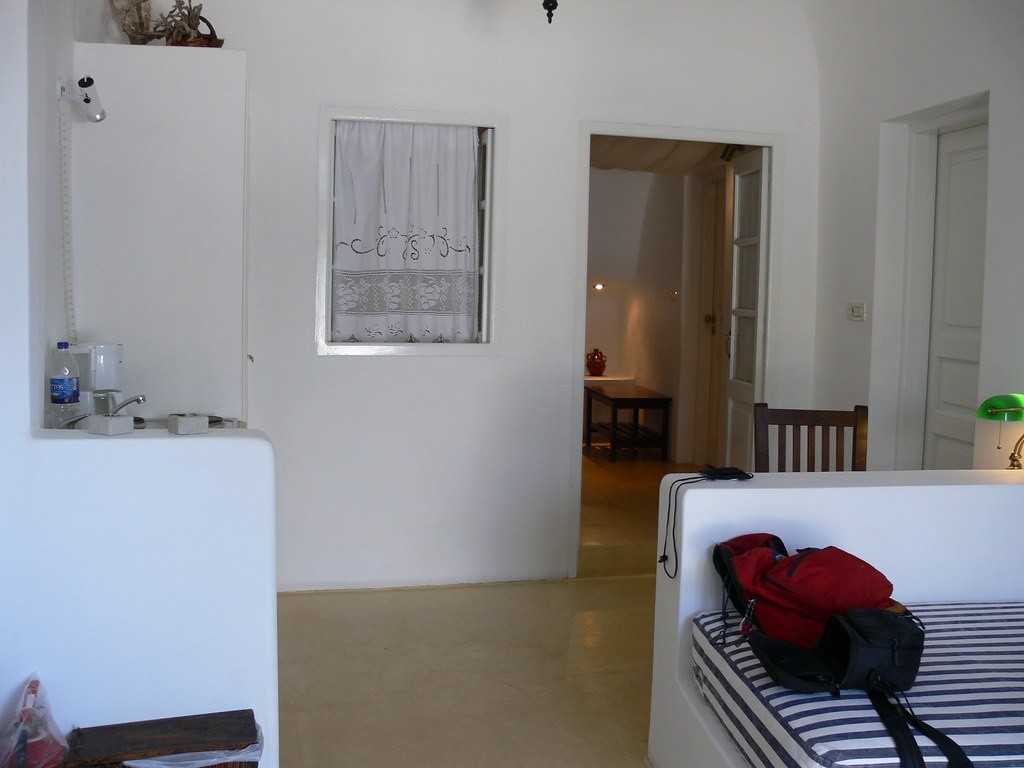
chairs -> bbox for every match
[753,402,868,472]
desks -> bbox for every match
[584,385,672,463]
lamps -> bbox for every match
[57,74,106,123]
[975,393,1024,470]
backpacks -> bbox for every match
[713,533,974,768]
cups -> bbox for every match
[73,343,127,415]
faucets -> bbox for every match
[104,390,146,416]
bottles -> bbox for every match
[50,342,80,429]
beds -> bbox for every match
[648,469,1024,768]
